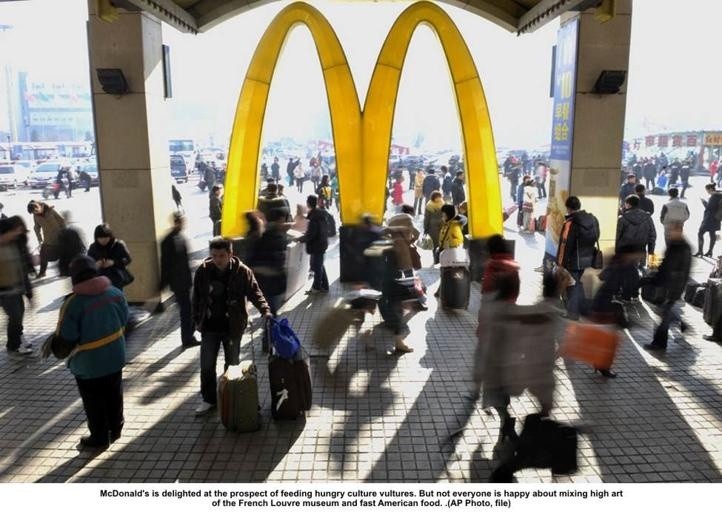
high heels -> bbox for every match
[594,363,617,378]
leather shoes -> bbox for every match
[182,336,202,348]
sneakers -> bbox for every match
[80,437,109,449]
[20,334,32,348]
[396,345,414,352]
[195,400,217,415]
[319,287,328,293]
[306,288,320,294]
[7,343,32,356]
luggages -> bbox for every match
[560,320,618,370]
[219,320,262,433]
[440,267,470,310]
[266,317,312,421]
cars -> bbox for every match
[170,137,230,181]
[388,152,432,170]
[0,155,100,194]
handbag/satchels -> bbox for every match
[410,246,421,270]
[433,246,442,264]
[106,266,134,288]
[317,197,328,207]
[691,287,706,308]
[416,234,434,249]
[685,283,705,302]
[592,250,603,269]
[271,317,301,358]
[439,247,470,268]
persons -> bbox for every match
[1,149,722,450]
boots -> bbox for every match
[692,237,703,257]
[704,236,716,257]
